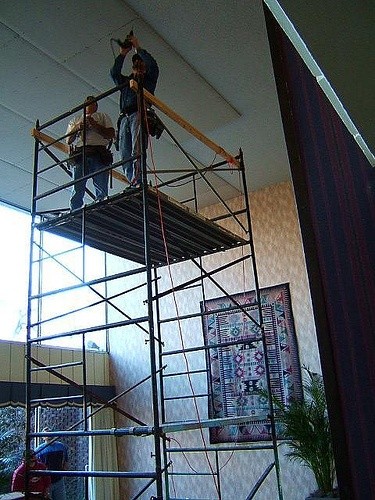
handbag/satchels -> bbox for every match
[145,108,164,140]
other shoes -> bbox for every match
[135,178,147,185]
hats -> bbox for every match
[132,53,142,62]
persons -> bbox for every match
[65,95,115,214]
[33,426,72,500]
[11,450,53,500]
[109,28,160,191]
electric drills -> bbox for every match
[115,27,135,51]
